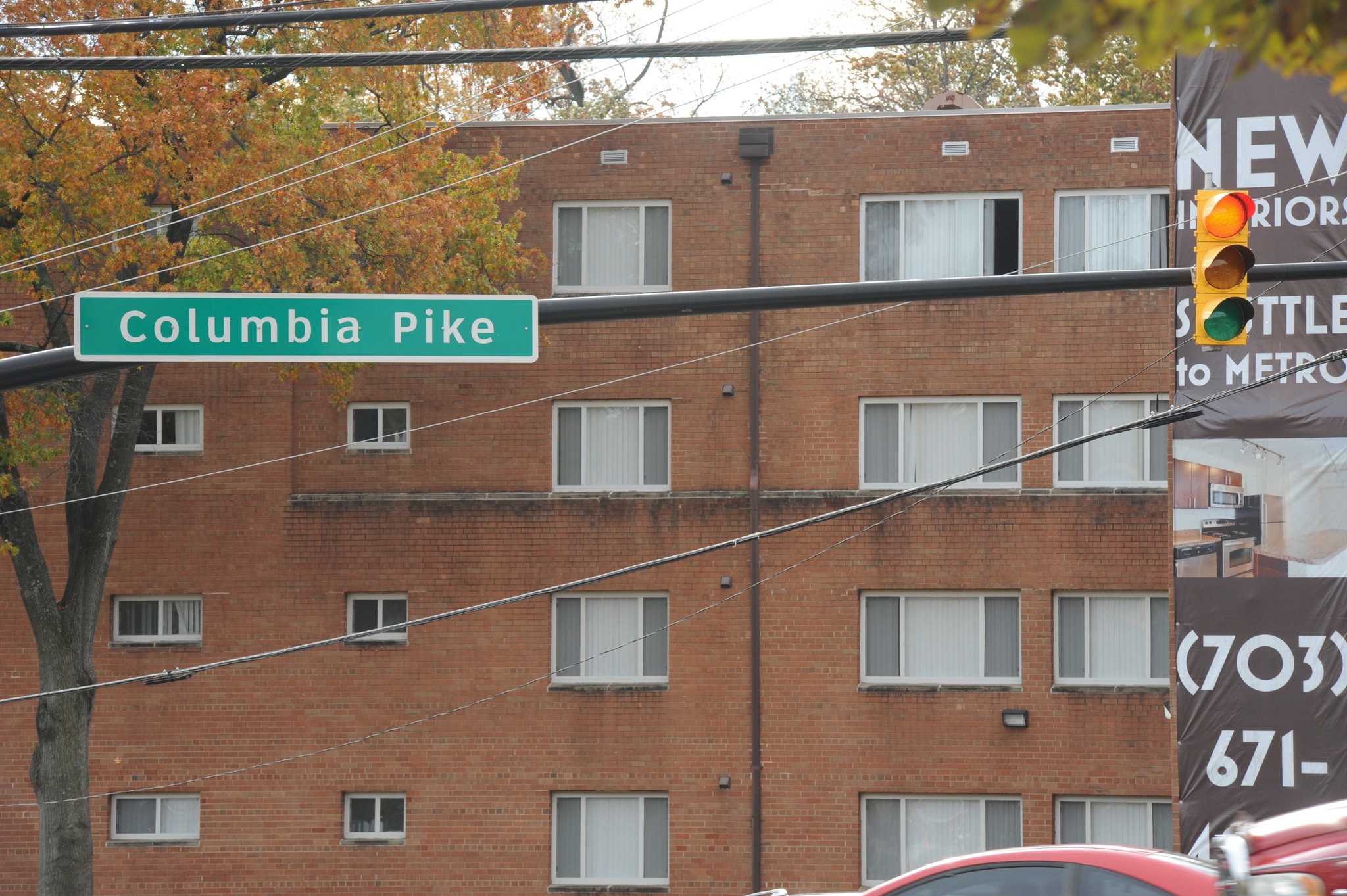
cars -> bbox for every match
[784,799,1347,896]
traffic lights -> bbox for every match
[1194,187,1258,344]
